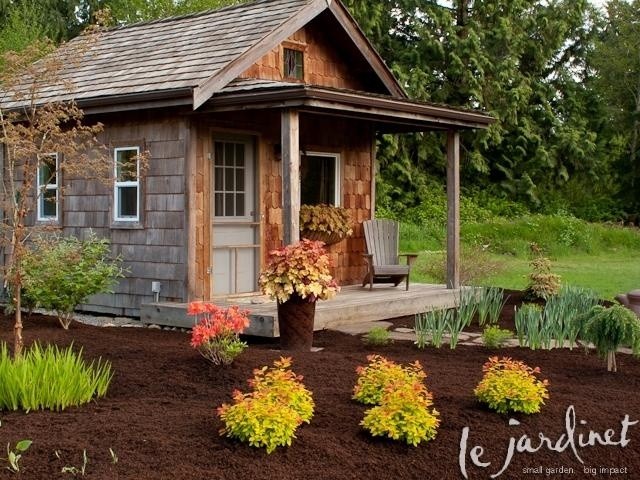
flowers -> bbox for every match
[185,300,251,365]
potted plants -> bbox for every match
[360,218,420,290]
[256,204,353,350]
